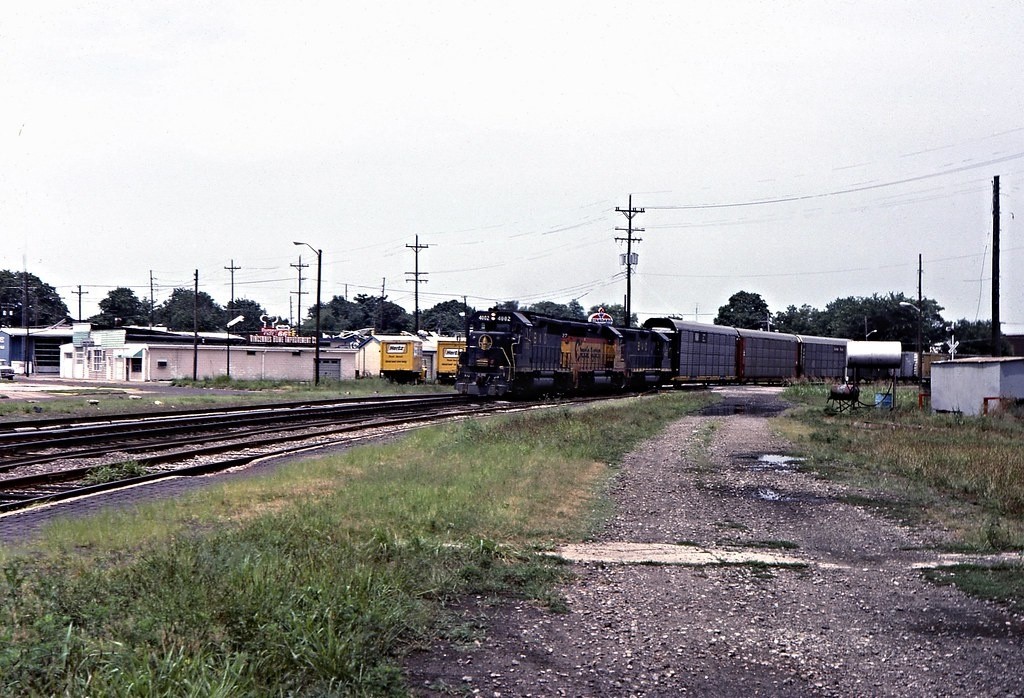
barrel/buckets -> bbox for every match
[875,393,891,410]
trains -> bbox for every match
[453,306,848,400]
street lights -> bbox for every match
[898,301,924,411]
[293,241,323,387]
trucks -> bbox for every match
[435,340,466,385]
[379,340,428,386]
